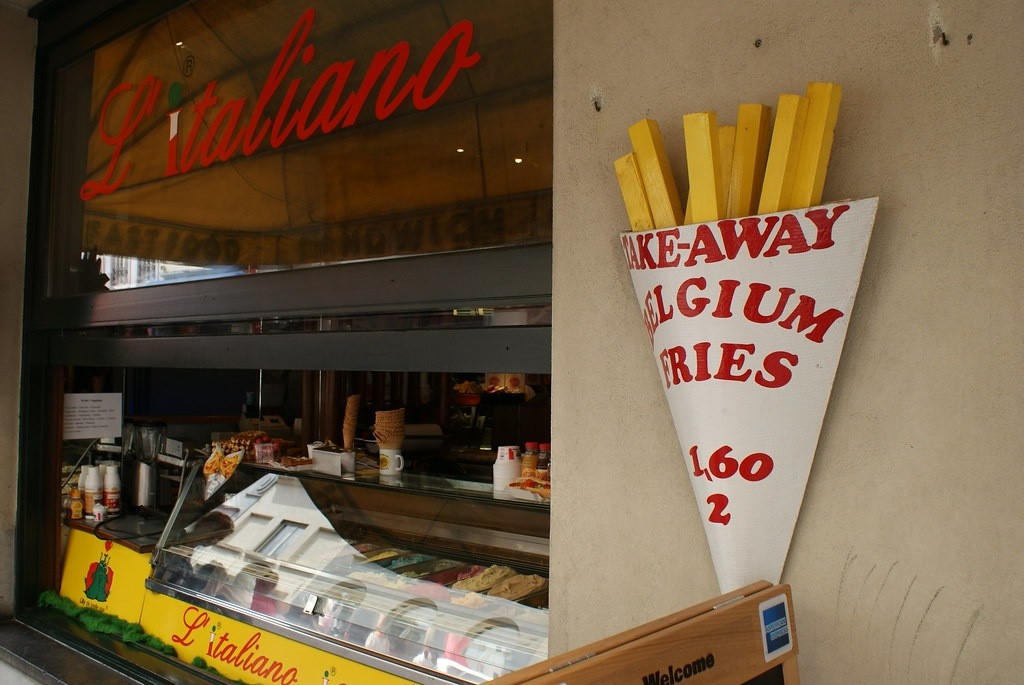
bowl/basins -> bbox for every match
[493,445,521,499]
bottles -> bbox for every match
[66,463,120,519]
[253,441,274,464]
[521,442,551,485]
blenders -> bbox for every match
[123,421,166,512]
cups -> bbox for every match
[92,508,108,521]
[378,448,404,475]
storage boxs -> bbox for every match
[312,446,356,478]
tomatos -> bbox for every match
[255,435,286,463]
